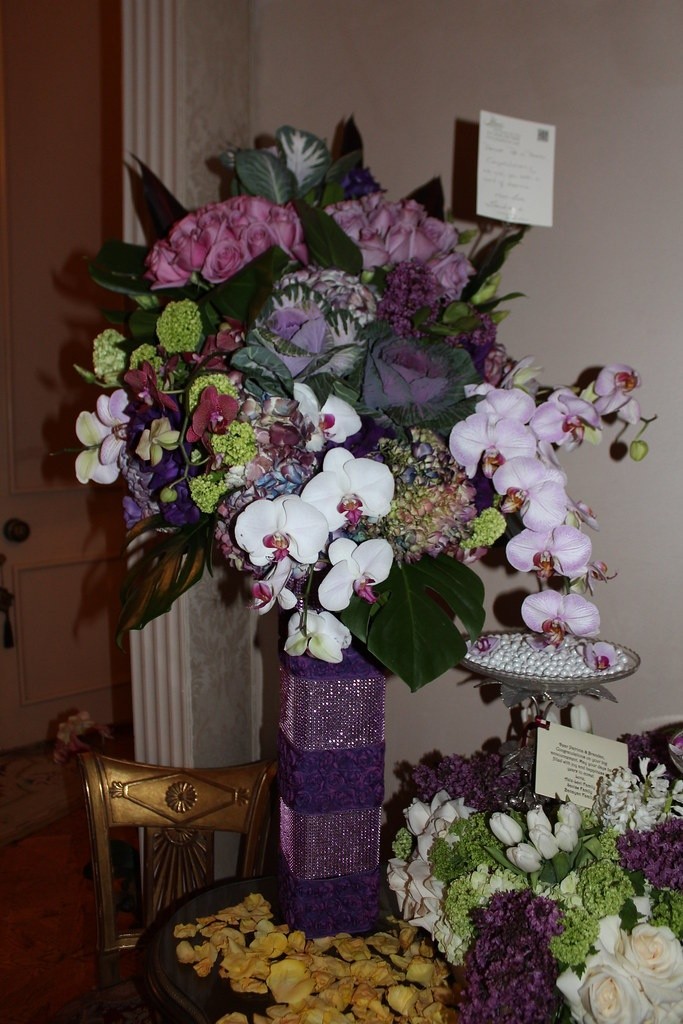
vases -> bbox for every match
[275,617,388,938]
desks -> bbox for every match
[152,875,336,1020]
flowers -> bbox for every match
[62,125,658,699]
[168,726,682,1024]
[43,712,142,923]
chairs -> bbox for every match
[46,754,274,1024]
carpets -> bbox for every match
[0,724,131,853]
